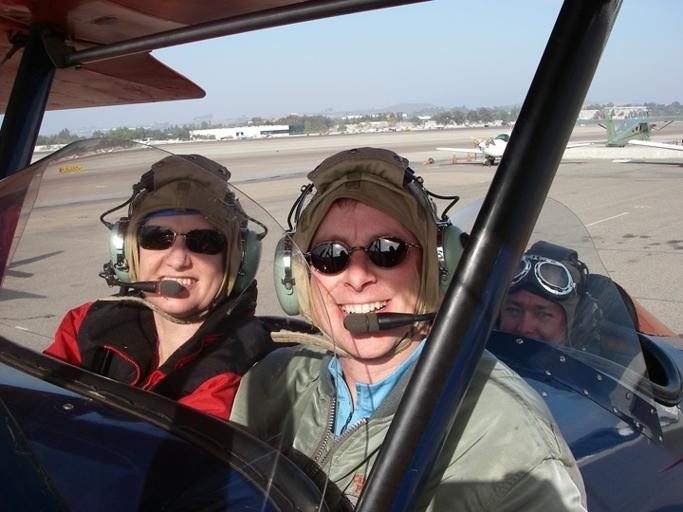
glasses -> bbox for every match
[504,255,581,302]
[298,233,421,277]
[134,224,230,257]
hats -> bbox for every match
[292,147,442,340]
[121,154,243,298]
[496,238,595,349]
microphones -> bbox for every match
[344,313,437,332]
[102,276,183,296]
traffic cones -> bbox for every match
[422,157,434,165]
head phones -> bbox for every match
[531,239,602,336]
[275,158,467,316]
[108,158,260,292]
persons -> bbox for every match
[498,240,609,355]
[41,155,297,423]
[228,148,588,512]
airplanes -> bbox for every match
[573,109,682,147]
[435,133,594,165]
[624,139,682,153]
